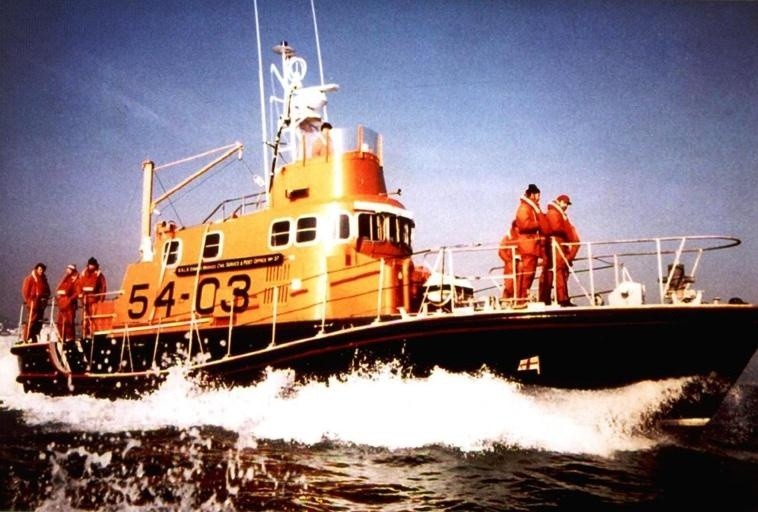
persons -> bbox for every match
[498,220,519,299]
[75,256,107,336]
[54,264,80,339]
[538,195,581,307]
[21,262,51,343]
[511,183,551,304]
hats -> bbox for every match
[67,264,77,269]
[524,184,541,195]
[557,196,574,205]
[36,263,45,269]
[88,257,98,267]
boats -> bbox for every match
[12,0,758,431]
